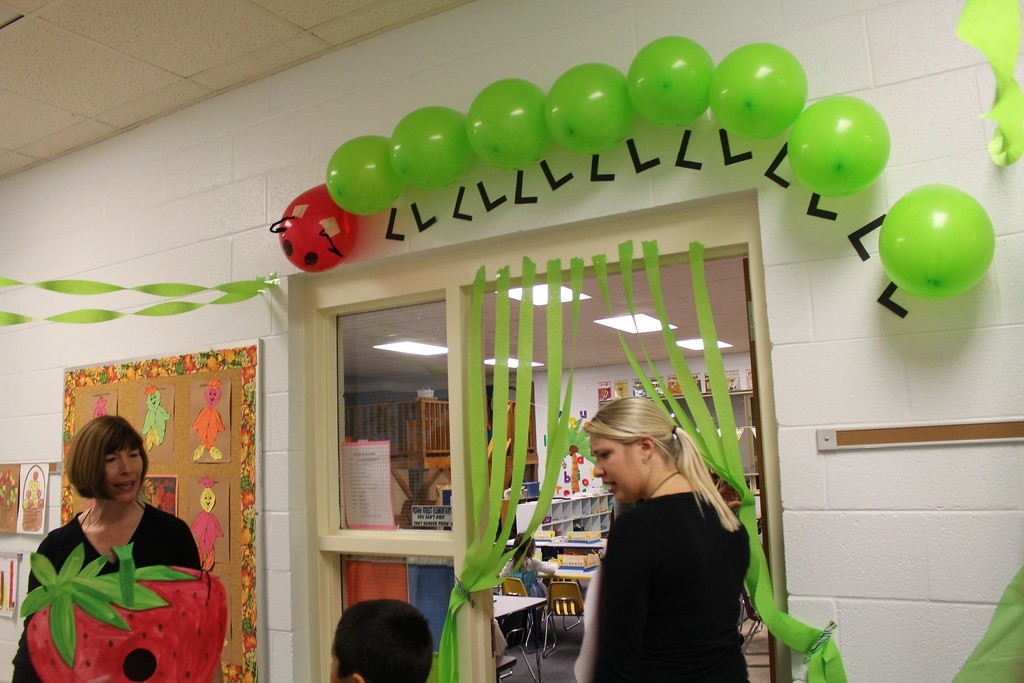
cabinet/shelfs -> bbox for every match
[515,492,616,539]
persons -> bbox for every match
[10,416,209,683]
[498,397,753,683]
[328,599,432,683]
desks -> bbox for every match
[492,535,608,555]
[493,593,547,683]
[538,568,598,631]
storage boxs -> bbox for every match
[523,481,539,496]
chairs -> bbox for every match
[735,577,770,668]
[494,578,585,683]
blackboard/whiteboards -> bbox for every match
[598,389,760,475]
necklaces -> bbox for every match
[650,470,681,498]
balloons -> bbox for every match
[268,36,994,301]
